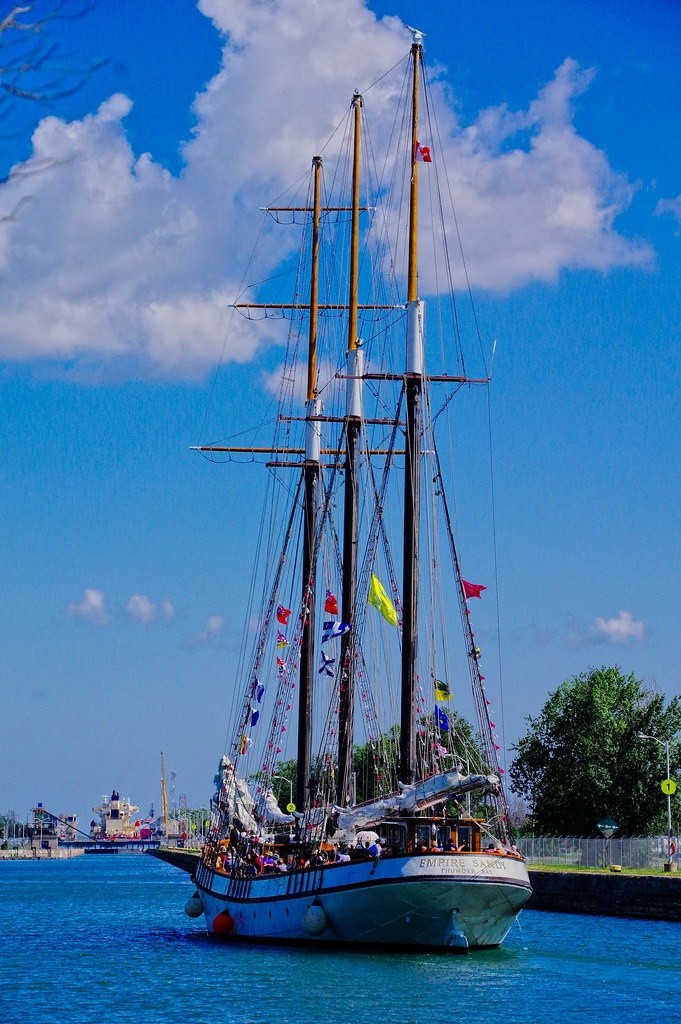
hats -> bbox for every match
[417,841,423,844]
[380,838,385,844]
[512,845,517,850]
[431,841,438,847]
[496,843,502,849]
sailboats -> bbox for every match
[189,26,535,958]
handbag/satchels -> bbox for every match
[368,845,378,856]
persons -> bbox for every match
[220,825,384,876]
[437,838,457,851]
[484,844,494,852]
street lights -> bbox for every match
[33,817,50,850]
[273,775,294,834]
[634,730,681,873]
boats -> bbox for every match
[143,751,204,875]
[525,855,583,868]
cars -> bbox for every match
[58,834,78,841]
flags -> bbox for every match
[368,571,398,627]
[438,745,448,758]
[247,707,259,727]
[252,681,265,703]
[322,621,352,645]
[276,630,291,648]
[460,578,487,600]
[277,657,286,665]
[239,734,253,754]
[435,732,441,746]
[434,678,453,701]
[434,705,451,731]
[325,589,338,615]
[415,141,432,162]
[277,604,292,625]
[318,650,336,678]
